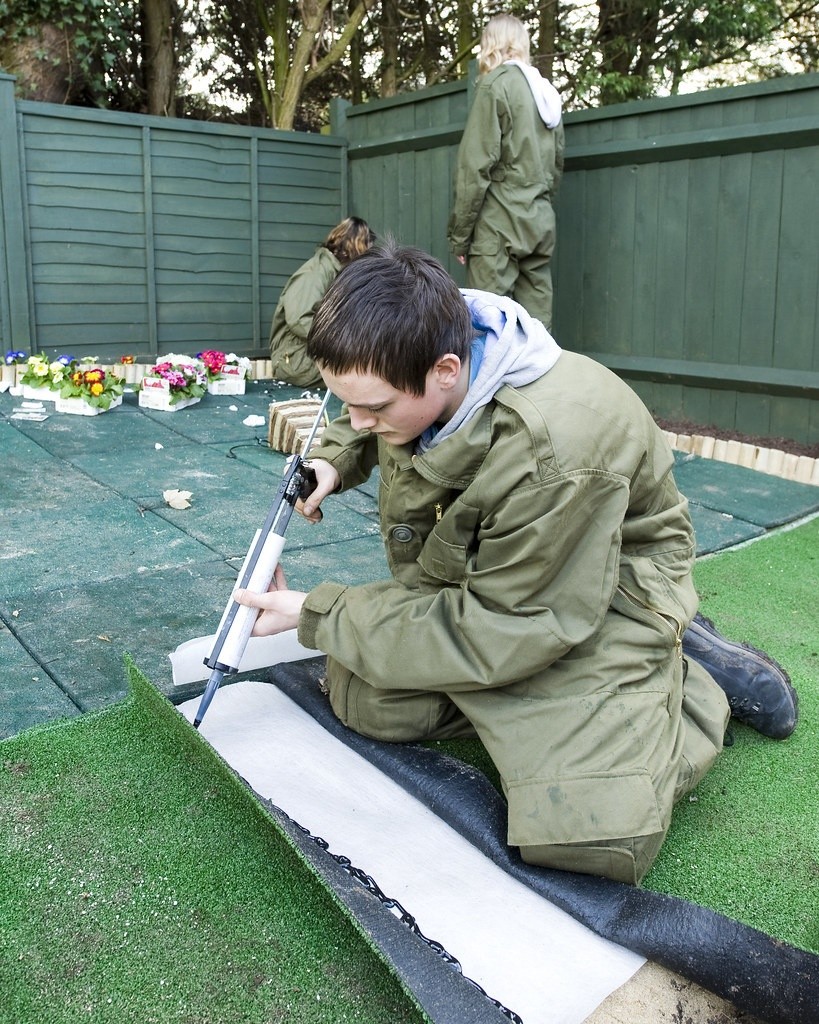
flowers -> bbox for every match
[0,350,135,410]
[227,353,252,382]
[148,350,207,405]
[197,350,225,382]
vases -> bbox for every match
[139,377,201,413]
[208,366,246,396]
[23,384,61,402]
[55,395,122,416]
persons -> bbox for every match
[445,12,566,333]
[231,232,799,890]
[269,216,377,390]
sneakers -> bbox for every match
[682,612,799,739]
